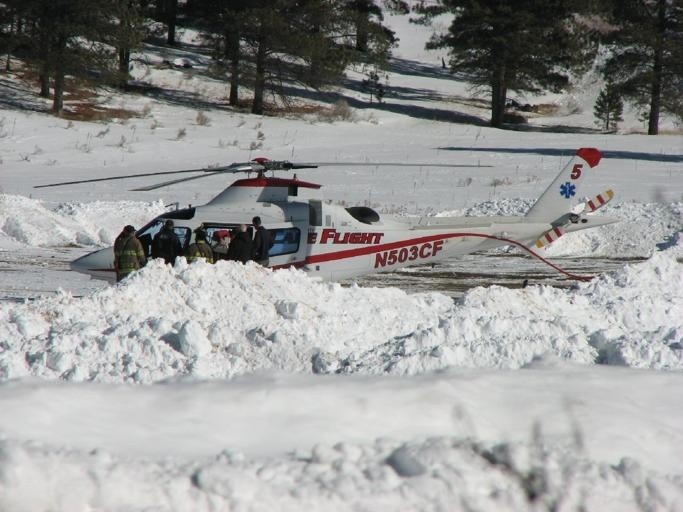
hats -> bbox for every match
[123,225,136,232]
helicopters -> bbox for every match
[32,148,622,283]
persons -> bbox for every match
[183,230,214,264]
[252,216,274,267]
[114,226,146,282]
[226,223,254,264]
[151,225,164,259]
[157,220,182,264]
